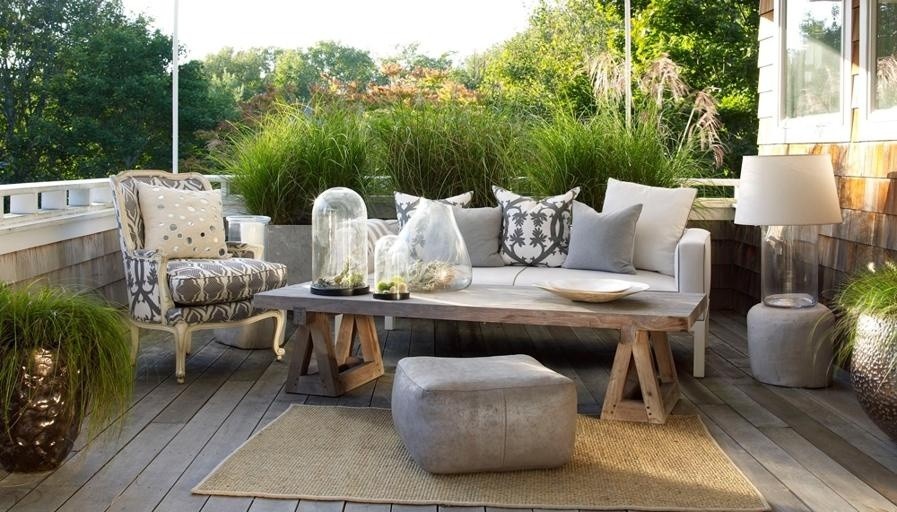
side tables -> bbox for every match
[746,305,834,393]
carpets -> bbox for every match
[192,404,772,511]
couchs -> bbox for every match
[333,217,712,381]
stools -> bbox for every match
[392,355,576,475]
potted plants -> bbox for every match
[0,280,134,474]
[809,255,896,443]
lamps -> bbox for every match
[734,154,844,309]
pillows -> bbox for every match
[395,190,477,230]
[397,198,509,267]
[134,179,227,259]
[560,200,642,277]
[492,184,582,268]
[600,177,697,276]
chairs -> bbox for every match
[111,170,287,382]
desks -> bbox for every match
[253,277,705,424]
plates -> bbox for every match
[553,279,632,294]
[533,278,650,303]
